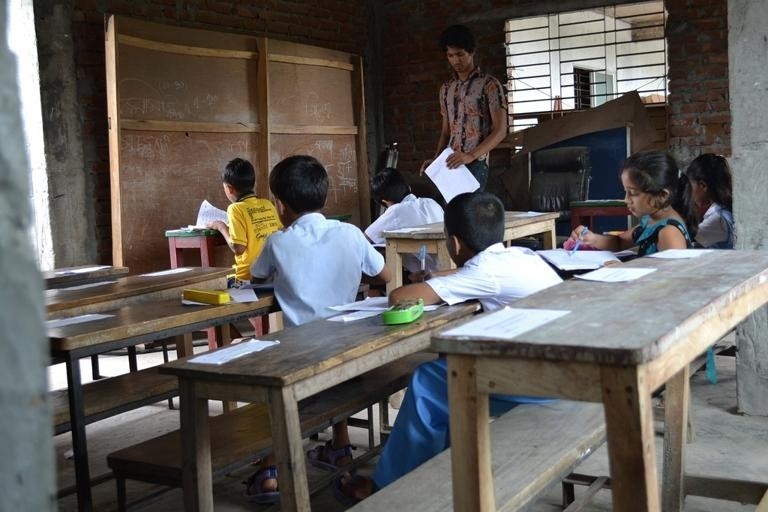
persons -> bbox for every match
[326,191,568,504]
[240,155,394,503]
[565,149,692,271]
[205,158,286,341]
[686,152,736,253]
[418,24,509,211]
[361,168,445,300]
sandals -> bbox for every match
[332,475,377,507]
[241,465,280,504]
[307,439,355,474]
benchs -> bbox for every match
[106,352,439,511]
[46,360,181,504]
[343,400,614,512]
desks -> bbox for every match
[573,202,643,233]
[48,265,139,380]
[431,255,767,512]
[157,296,483,512]
[49,267,237,439]
[48,288,281,512]
[381,210,563,408]
[166,227,271,352]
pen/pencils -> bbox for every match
[419,245,425,283]
[571,225,589,255]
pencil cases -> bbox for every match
[383,297,425,325]
[184,288,230,305]
